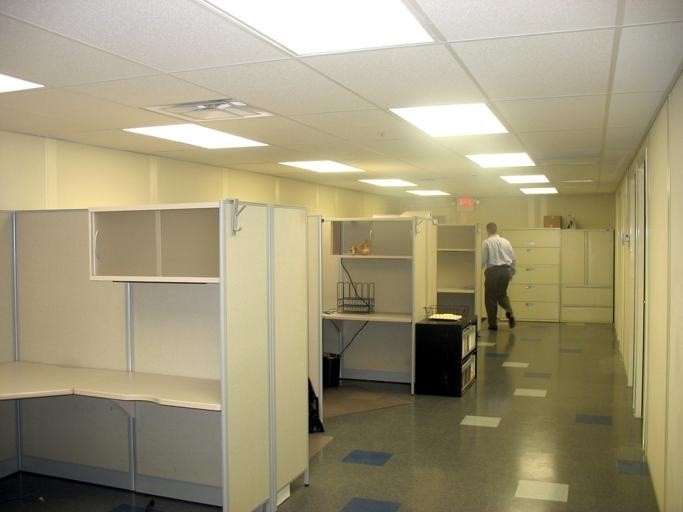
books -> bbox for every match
[460,325,475,390]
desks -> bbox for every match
[436,290,478,323]
[321,313,425,395]
[0,363,273,512]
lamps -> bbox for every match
[520,187,558,195]
[502,175,550,184]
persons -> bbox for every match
[481,223,516,329]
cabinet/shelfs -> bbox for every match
[613,145,644,419]
[503,228,561,323]
[643,75,683,512]
[560,228,613,324]
[414,314,478,398]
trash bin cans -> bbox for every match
[323,353,340,388]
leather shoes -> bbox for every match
[488,326,497,330]
[510,316,514,328]
